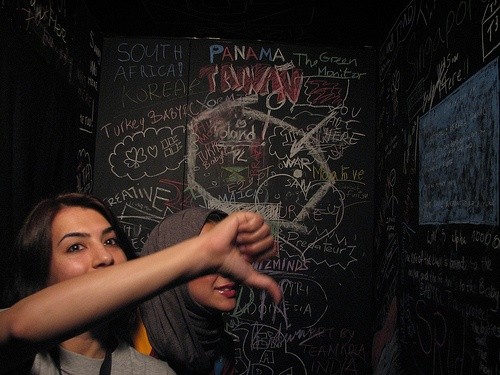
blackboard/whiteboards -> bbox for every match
[375,0,500,375]
[86,35,380,375]
[0,0,103,199]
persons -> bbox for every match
[0,191,283,374]
[126,208,242,375]
